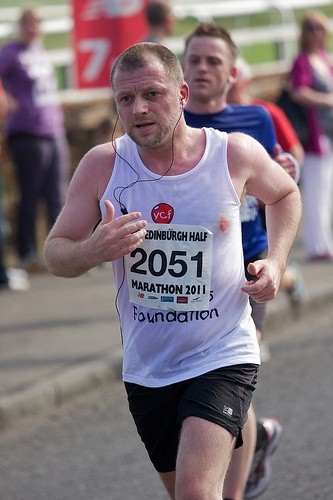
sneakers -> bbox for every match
[286,262,307,320]
[245,416,281,499]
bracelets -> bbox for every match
[281,153,300,184]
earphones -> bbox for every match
[181,95,184,101]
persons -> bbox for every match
[182,21,299,500]
[287,11,333,261]
[142,4,174,49]
[228,57,305,310]
[44,42,303,500]
[0,10,70,269]
